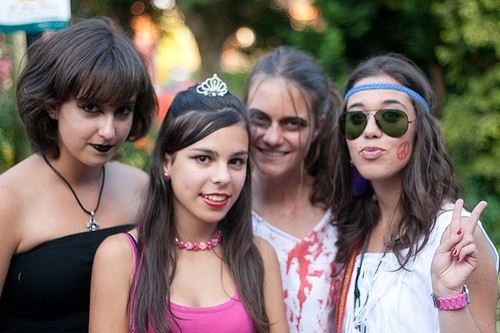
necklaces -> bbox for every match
[354,228,393,333]
[172,229,222,250]
[42,153,106,231]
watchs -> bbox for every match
[431,284,470,311]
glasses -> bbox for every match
[338,109,416,140]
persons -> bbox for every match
[244,47,345,333]
[326,54,499,333]
[0,17,160,333]
[88,72,290,333]
